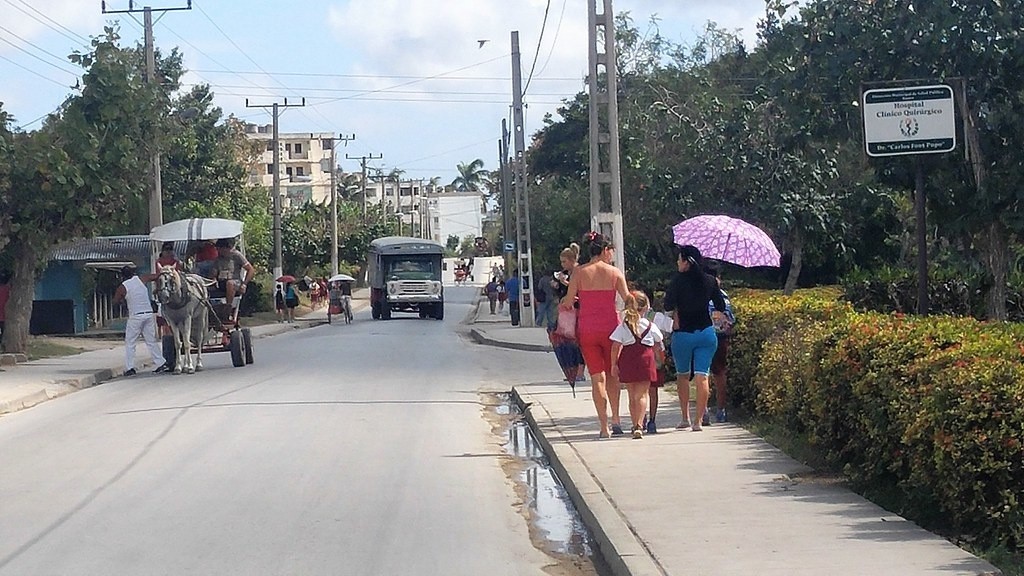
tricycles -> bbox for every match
[326,274,355,324]
[453,267,467,286]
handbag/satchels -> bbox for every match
[555,301,578,341]
[709,288,735,333]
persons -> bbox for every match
[535,270,555,327]
[154,295,168,340]
[0,268,11,343]
[484,276,500,315]
[273,284,287,323]
[551,243,586,381]
[156,243,178,283]
[339,280,351,296]
[212,238,254,321]
[112,265,170,376]
[664,245,726,431]
[562,229,630,440]
[492,262,504,277]
[284,282,298,323]
[307,278,321,312]
[504,268,527,327]
[607,258,736,439]
[496,281,507,310]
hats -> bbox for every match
[121,266,136,278]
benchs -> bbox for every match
[209,272,246,306]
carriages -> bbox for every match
[149,218,254,376]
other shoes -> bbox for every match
[692,424,702,430]
[630,425,643,439]
[676,420,691,429]
[716,409,726,422]
[611,427,625,437]
[702,412,710,425]
[647,423,656,434]
[564,376,586,381]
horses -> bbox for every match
[156,261,210,375]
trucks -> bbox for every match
[369,237,447,322]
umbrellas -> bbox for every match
[672,215,782,280]
[547,327,586,399]
[275,275,296,283]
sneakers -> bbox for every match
[124,368,137,378]
[152,364,169,375]
